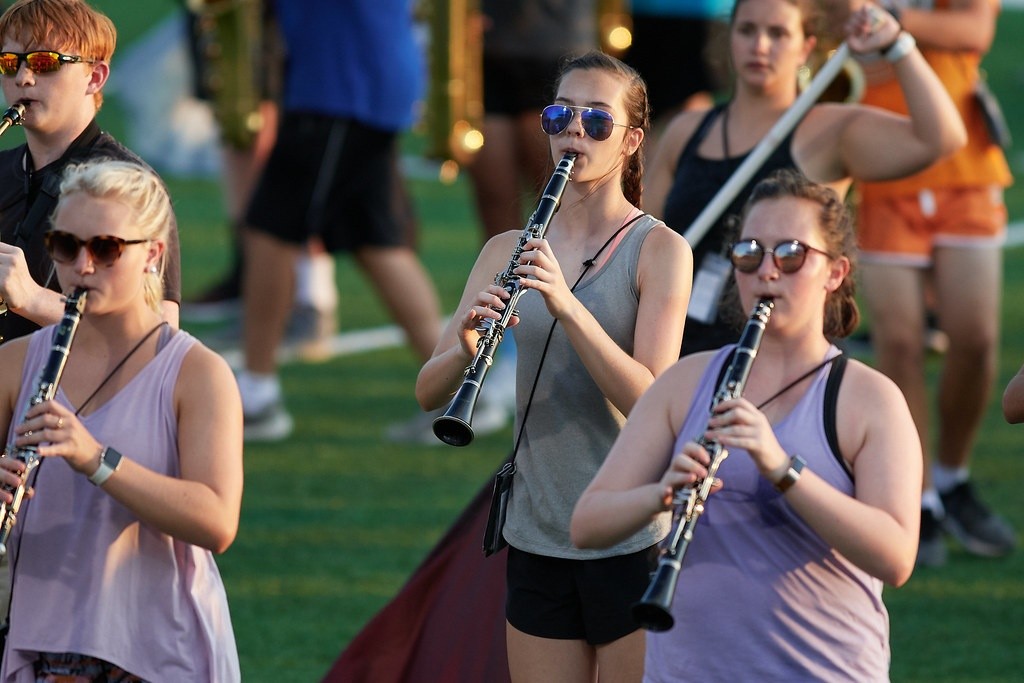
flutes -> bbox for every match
[631,295,775,634]
[432,149,579,449]
[0,97,31,136]
[0,285,90,562]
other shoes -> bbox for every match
[182,264,247,320]
[276,302,340,352]
[236,372,293,441]
[387,380,509,445]
[917,471,1017,572]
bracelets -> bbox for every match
[774,452,807,496]
[880,28,904,57]
[886,33,916,66]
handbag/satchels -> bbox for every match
[970,83,1014,150]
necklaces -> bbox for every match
[569,170,923,682]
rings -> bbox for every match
[55,417,64,429]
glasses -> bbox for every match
[39,228,151,270]
[538,103,637,143]
[726,238,835,274]
[0,51,91,77]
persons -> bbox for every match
[468,0,597,243]
[0,161,243,683]
[182,0,335,365]
[644,0,968,354]
[853,0,1023,569]
[416,53,697,683]
[0,1,181,343]
[243,0,442,449]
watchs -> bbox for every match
[89,445,122,487]
